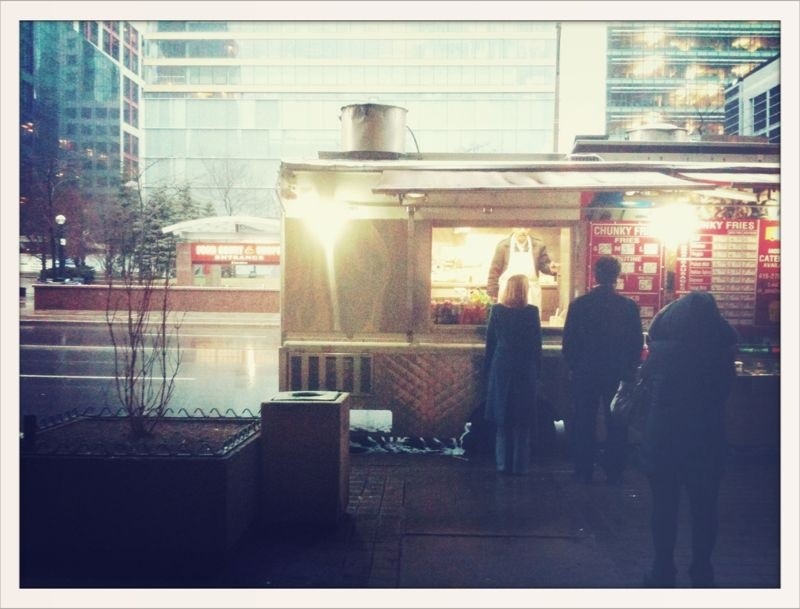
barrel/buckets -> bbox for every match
[340,104,408,152]
[625,127,687,141]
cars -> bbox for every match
[38,266,97,284]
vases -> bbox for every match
[20,404,266,589]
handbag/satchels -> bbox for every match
[608,377,643,420]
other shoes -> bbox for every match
[605,473,619,487]
[645,573,676,588]
[577,470,592,487]
[512,462,541,477]
[496,461,512,473]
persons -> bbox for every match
[488,226,561,321]
[480,275,543,476]
[560,253,643,488]
[637,290,741,585]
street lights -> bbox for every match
[55,213,68,268]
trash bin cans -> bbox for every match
[260,390,352,529]
